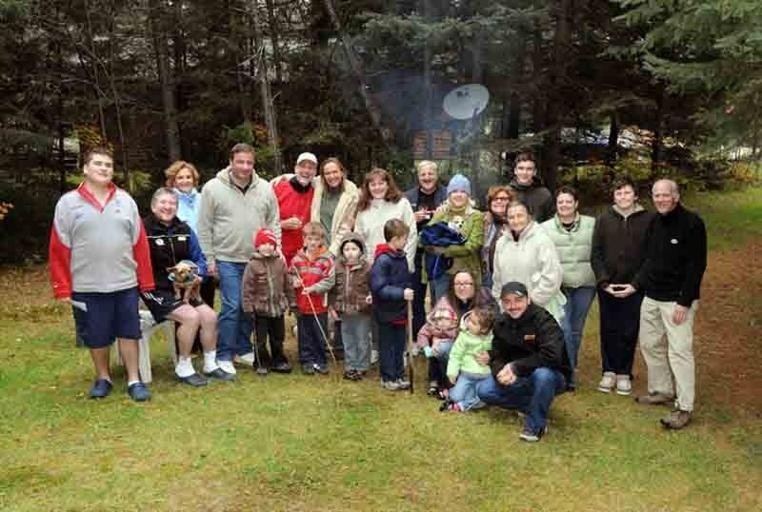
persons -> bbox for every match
[166,142,596,411]
[48,144,153,403]
[136,186,234,386]
[634,179,708,427]
[591,176,653,396]
[477,282,575,443]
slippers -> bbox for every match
[127,381,152,402]
[173,371,209,387]
[89,379,114,399]
[202,368,236,381]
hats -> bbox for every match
[253,228,277,250]
[341,239,364,257]
[295,151,319,165]
[446,173,472,196]
[500,282,528,297]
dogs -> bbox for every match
[448,215,468,237]
[166,260,202,304]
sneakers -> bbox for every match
[427,382,438,396]
[659,408,693,430]
[314,363,331,375]
[378,376,401,391]
[256,367,270,376]
[301,361,315,375]
[354,369,369,381]
[232,351,255,367]
[216,359,237,375]
[614,373,633,396]
[513,409,529,420]
[369,349,380,365]
[438,387,450,400]
[393,377,410,391]
[342,368,357,381]
[634,392,678,407]
[274,362,293,374]
[597,371,618,393]
[518,424,548,442]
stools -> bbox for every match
[107,312,177,381]
